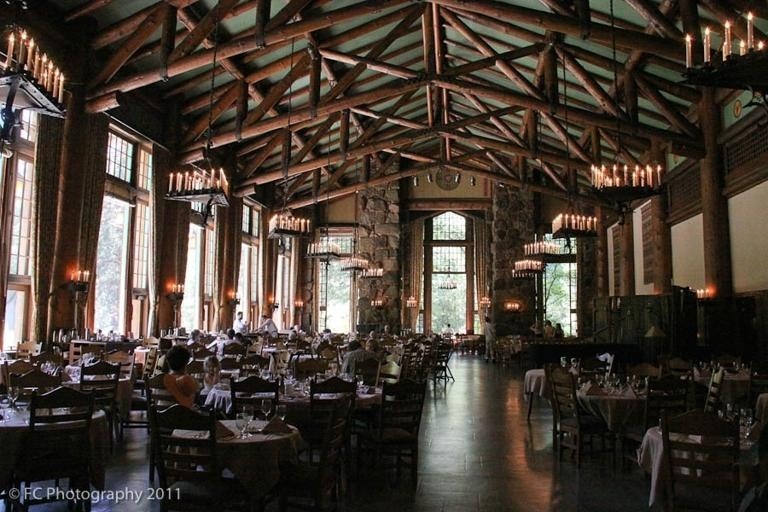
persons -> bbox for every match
[444,324,454,335]
[484,317,497,363]
[530,320,564,338]
[163,312,399,413]
[580,322,592,338]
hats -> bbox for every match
[162,372,200,410]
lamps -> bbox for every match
[1,0,384,312]
[499,0,768,283]
[409,170,476,188]
[438,212,456,289]
[694,286,716,308]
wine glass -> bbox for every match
[718,403,757,445]
[1,327,424,439]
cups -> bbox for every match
[555,354,764,398]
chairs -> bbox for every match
[0,331,766,512]
